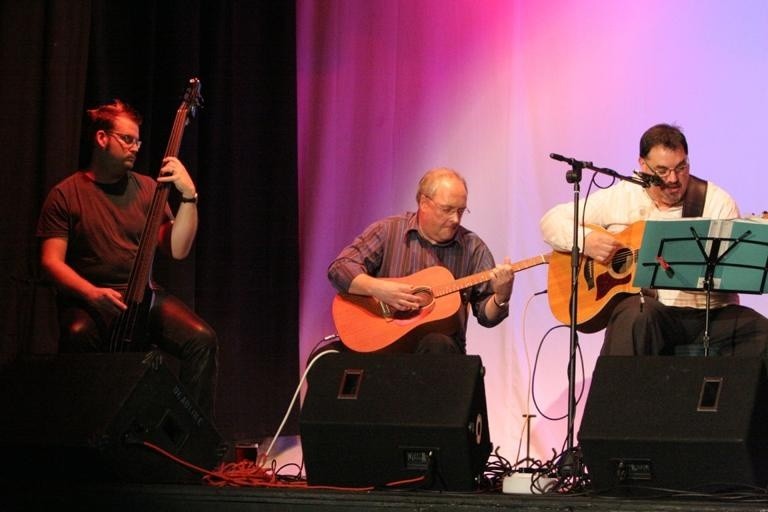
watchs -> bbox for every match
[490,296,510,308]
[178,194,201,204]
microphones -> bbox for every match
[633,169,663,186]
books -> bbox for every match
[631,214,768,297]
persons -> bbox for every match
[304,164,513,384]
[26,92,225,447]
[537,120,767,361]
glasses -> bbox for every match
[424,193,471,215]
[109,131,142,148]
[645,160,689,177]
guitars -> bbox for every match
[110,76,209,352]
[332,252,551,354]
[547,211,768,334]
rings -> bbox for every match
[169,167,173,173]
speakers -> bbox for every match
[576,355,767,494]
[1,351,228,484]
[298,353,492,489]
